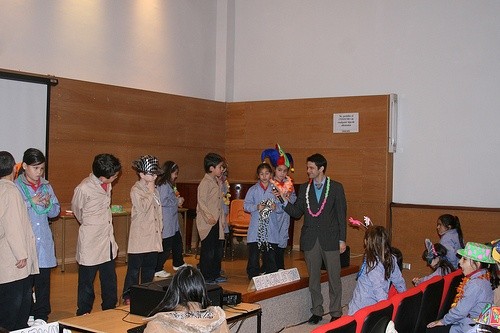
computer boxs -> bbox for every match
[129,276,223,317]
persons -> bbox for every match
[0,151,40,332]
[155,160,193,278]
[435,214,466,271]
[71,172,120,316]
[121,156,164,306]
[143,265,233,333]
[261,143,297,276]
[385,242,496,333]
[412,238,457,287]
[272,152,347,324]
[13,148,61,324]
[194,168,231,276]
[349,225,407,315]
[243,162,286,279]
[470,236,500,333]
[196,152,229,286]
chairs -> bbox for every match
[310,316,357,333]
[434,269,466,333]
[227,199,251,263]
[390,287,423,333]
[413,276,445,333]
[352,299,394,333]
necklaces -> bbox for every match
[450,273,491,310]
[306,176,331,217]
[219,180,231,205]
[18,173,53,215]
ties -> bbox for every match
[315,183,323,203]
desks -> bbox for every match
[58,302,263,332]
[49,205,189,273]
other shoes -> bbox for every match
[385,321,398,333]
[154,270,171,277]
[173,263,193,270]
[206,269,285,285]
[27,316,47,327]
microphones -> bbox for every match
[270,183,285,206]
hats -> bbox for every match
[455,241,498,264]
[131,155,165,175]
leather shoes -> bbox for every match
[329,317,341,322]
[308,314,322,325]
